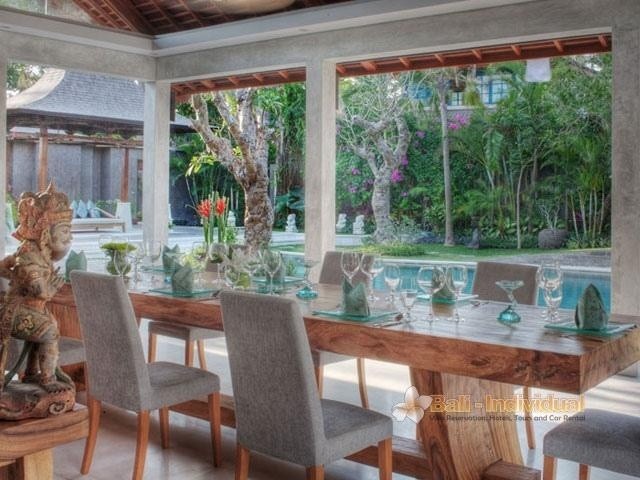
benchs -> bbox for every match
[70,208,126,235]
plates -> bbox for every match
[545,320,634,337]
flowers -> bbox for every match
[196,193,231,244]
[391,151,409,183]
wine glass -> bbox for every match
[491,279,526,322]
[535,258,563,322]
[418,264,446,321]
[339,250,420,322]
[99,234,320,299]
[446,263,468,322]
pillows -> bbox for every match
[70,199,102,218]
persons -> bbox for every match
[0,181,73,390]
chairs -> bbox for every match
[69,247,640,480]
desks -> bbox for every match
[44,272,638,479]
